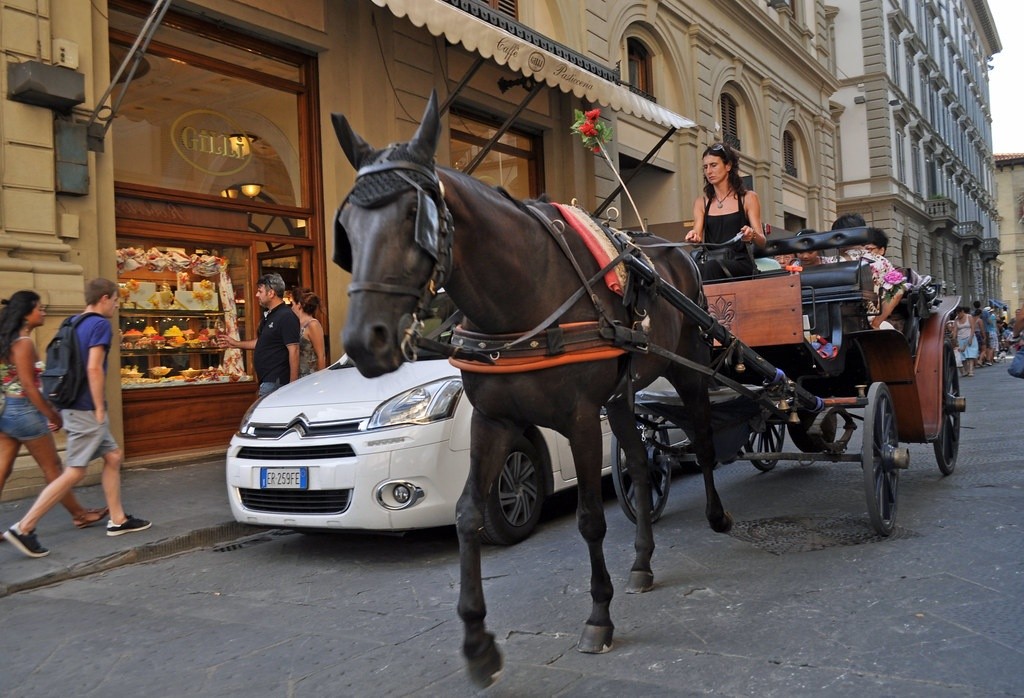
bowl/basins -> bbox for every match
[179,370,206,378]
[148,367,173,376]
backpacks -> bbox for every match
[40,312,112,409]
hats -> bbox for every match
[984,307,994,312]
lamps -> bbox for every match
[240,182,264,199]
[228,134,256,158]
[220,187,240,199]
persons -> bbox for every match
[3,277,152,558]
[290,287,325,378]
[946,301,1024,376]
[0,290,112,542]
[685,143,766,285]
[772,212,907,331]
[217,273,300,397]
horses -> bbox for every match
[330,88,734,690]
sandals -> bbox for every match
[72,506,109,529]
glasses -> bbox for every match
[866,247,878,252]
[707,143,727,156]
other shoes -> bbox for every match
[986,362,992,366]
[962,372,973,377]
[977,360,983,367]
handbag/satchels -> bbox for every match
[698,248,749,262]
[1008,346,1024,379]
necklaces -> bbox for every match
[714,189,733,209]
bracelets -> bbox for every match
[1012,334,1020,339]
[752,227,756,241]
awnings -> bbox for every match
[989,299,1007,310]
[364,0,698,219]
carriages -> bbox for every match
[331,91,969,694]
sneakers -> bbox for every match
[106,514,151,536]
[3,522,51,558]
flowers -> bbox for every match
[571,109,613,154]
[883,268,906,290]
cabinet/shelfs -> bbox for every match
[118,306,256,393]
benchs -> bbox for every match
[689,225,875,305]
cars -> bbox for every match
[226,288,755,546]
